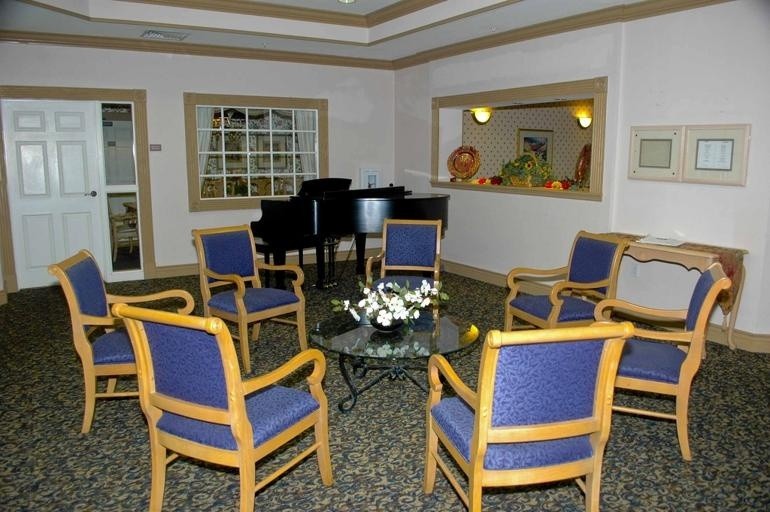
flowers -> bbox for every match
[331,280,449,327]
[501,151,551,191]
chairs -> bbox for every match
[589,262,732,460]
[191,224,307,375]
[48,249,195,434]
[108,197,139,262]
[504,230,629,332]
[366,218,441,314]
[424,323,636,512]
[112,301,333,512]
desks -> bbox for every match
[594,233,749,353]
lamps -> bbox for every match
[578,117,592,128]
[474,113,491,125]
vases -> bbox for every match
[369,319,404,332]
[509,175,543,187]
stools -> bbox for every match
[322,242,339,282]
[255,242,304,288]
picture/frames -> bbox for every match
[682,124,751,187]
[360,167,383,189]
[627,125,686,183]
[216,134,247,170]
[257,134,287,169]
[517,128,554,168]
